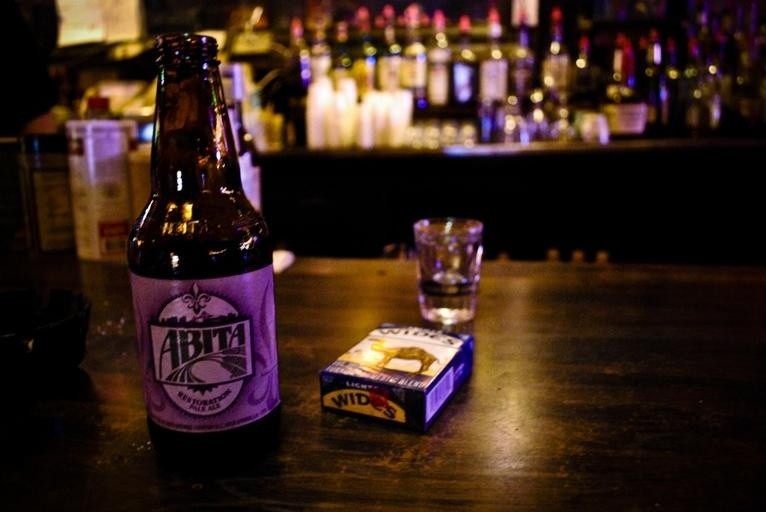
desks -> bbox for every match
[250,138,726,218]
[69,257,766,511]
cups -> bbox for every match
[414,219,484,325]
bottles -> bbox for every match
[129,32,284,476]
[451,15,477,104]
[478,5,507,101]
[540,7,570,89]
[82,96,115,118]
[376,5,402,92]
[22,132,74,251]
[599,35,648,138]
[224,62,260,211]
[68,119,135,263]
[647,42,669,126]
[508,10,534,93]
[288,19,310,95]
[402,4,425,105]
[310,16,330,77]
[330,22,351,87]
[352,7,375,98]
[426,10,451,106]
